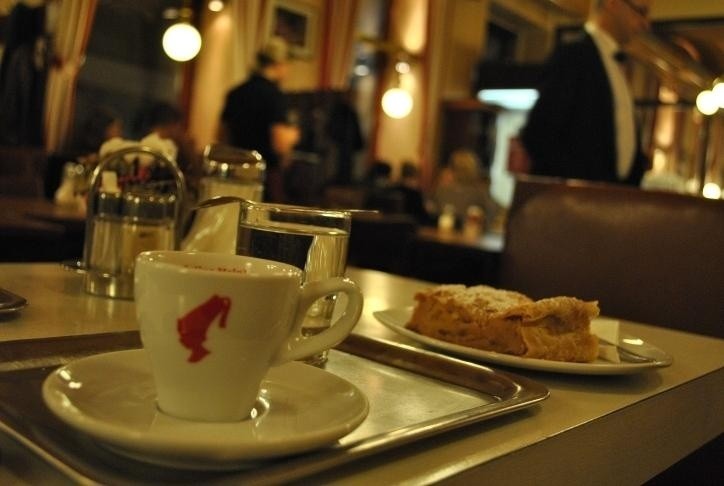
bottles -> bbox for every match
[52,161,85,212]
[198,140,269,233]
[88,186,178,304]
[437,203,455,239]
[464,204,484,244]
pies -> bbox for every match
[404,283,600,363]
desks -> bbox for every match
[2,129,508,286]
[1,262,723,485]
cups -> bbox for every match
[133,249,364,421]
[237,200,350,369]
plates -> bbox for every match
[375,305,678,375]
[42,347,373,475]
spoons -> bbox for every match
[185,196,383,214]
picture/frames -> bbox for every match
[256,0,321,65]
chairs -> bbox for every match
[505,173,723,485]
